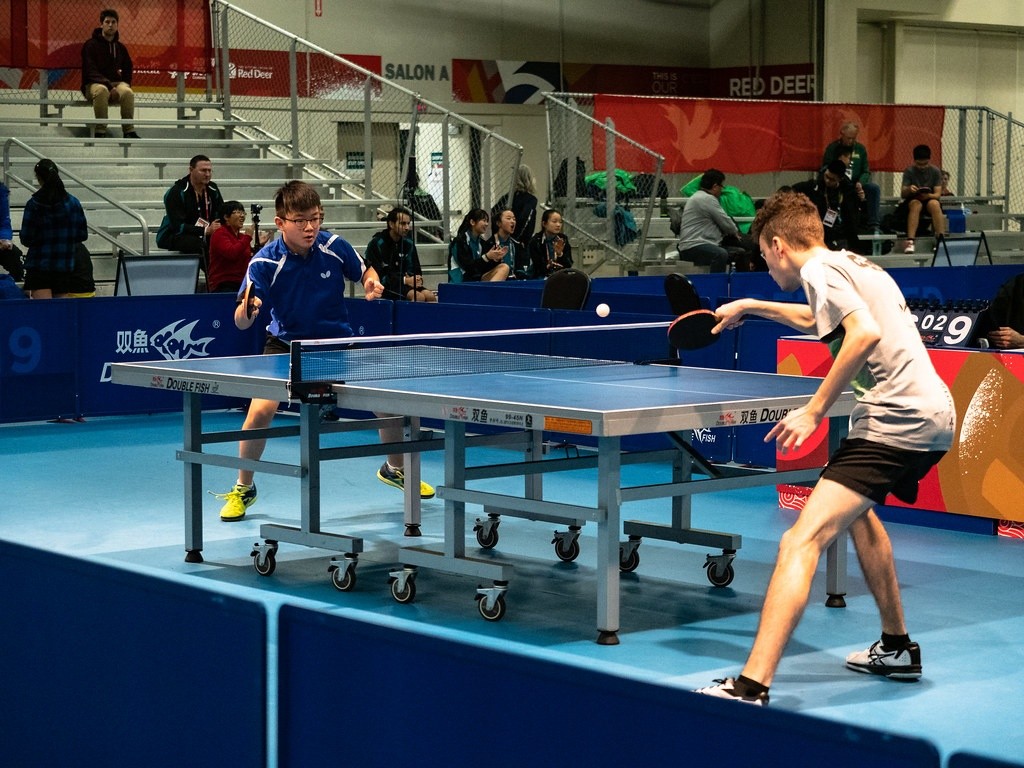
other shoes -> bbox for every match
[94,132,105,138]
[123,131,140,138]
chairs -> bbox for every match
[664,274,702,318]
[540,268,592,310]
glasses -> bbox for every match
[718,183,725,190]
[278,216,323,229]
[760,245,775,261]
[232,211,247,217]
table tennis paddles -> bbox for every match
[666,309,751,350]
[916,186,932,193]
[243,280,257,320]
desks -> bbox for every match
[107,335,859,646]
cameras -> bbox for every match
[250,204,263,214]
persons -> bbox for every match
[447,209,509,281]
[20,159,96,298]
[0,182,25,281]
[365,209,434,302]
[527,210,573,278]
[155,155,224,273]
[901,144,952,254]
[220,181,436,522]
[969,272,1024,350]
[488,209,528,280]
[790,120,880,251]
[208,201,274,292]
[491,164,537,243]
[680,168,751,274]
[81,9,141,139]
[687,192,957,710]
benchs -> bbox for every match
[555,193,1024,279]
[0,99,462,296]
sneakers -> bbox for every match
[694,677,773,707]
[206,481,258,522]
[377,461,436,500]
[845,639,924,677]
[904,240,915,253]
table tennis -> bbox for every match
[595,303,611,318]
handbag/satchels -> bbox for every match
[75,241,96,292]
